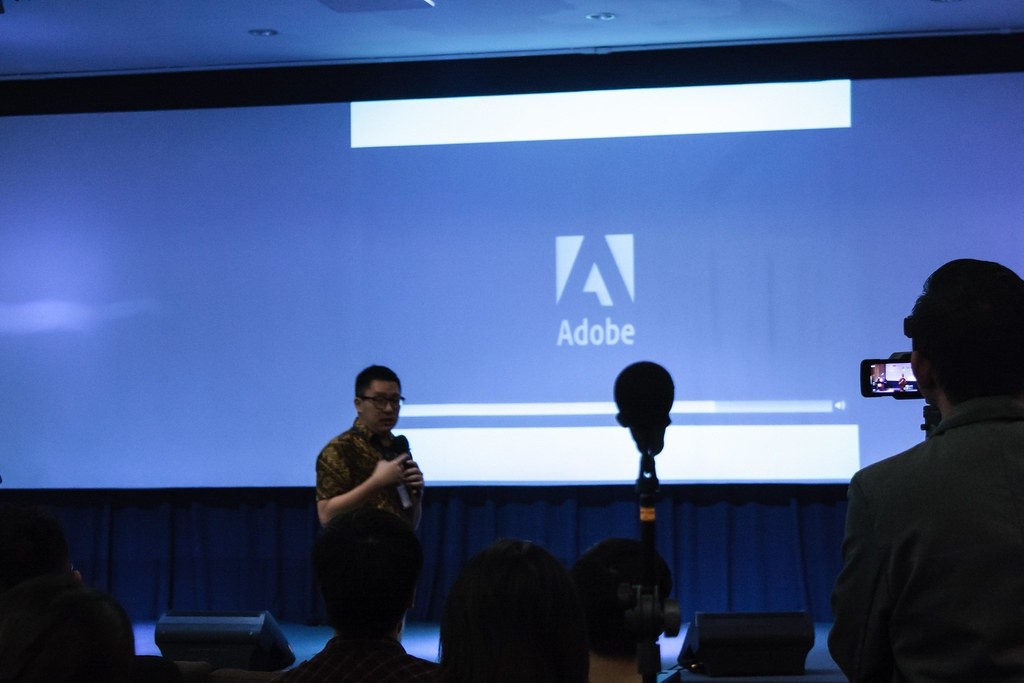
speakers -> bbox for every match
[154,611,296,672]
[677,612,814,677]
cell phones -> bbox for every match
[860,359,919,398]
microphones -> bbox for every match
[395,435,421,497]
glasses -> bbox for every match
[357,394,406,409]
[900,310,965,346]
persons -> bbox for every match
[272,509,441,683]
[315,364,424,643]
[899,374,906,390]
[437,538,586,683]
[568,537,673,683]
[0,506,183,683]
[827,258,1024,683]
